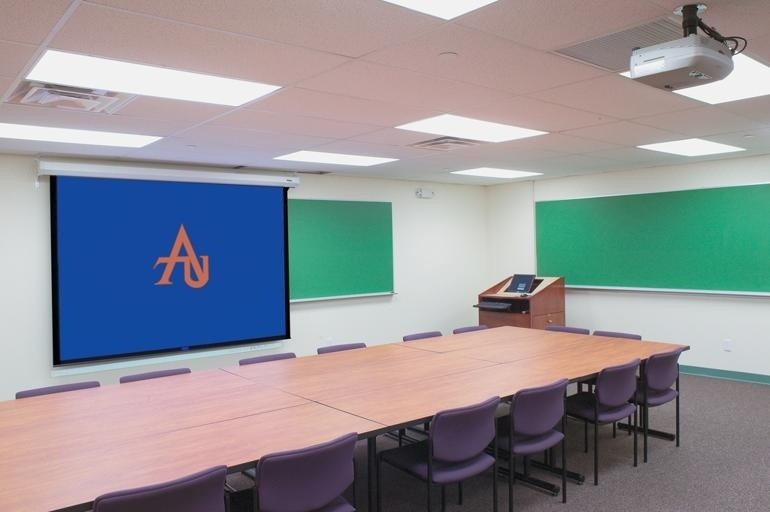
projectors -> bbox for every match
[629,34,734,92]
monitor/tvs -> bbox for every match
[507,274,536,293]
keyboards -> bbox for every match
[478,301,512,309]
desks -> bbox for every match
[1,366,387,512]
[220,325,690,444]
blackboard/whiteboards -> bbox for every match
[534,183,770,296]
[288,199,397,303]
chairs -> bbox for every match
[228,431,359,511]
[91,463,229,511]
[457,378,572,510]
[561,359,642,486]
[374,396,502,510]
[612,347,684,464]
